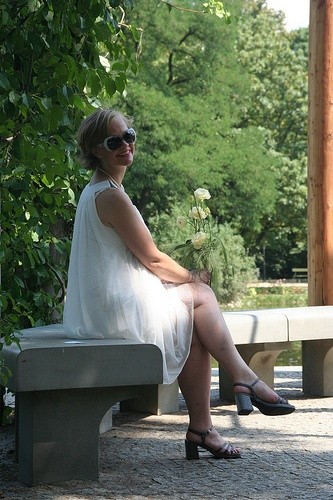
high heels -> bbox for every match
[233,377,296,416]
[185,425,242,461]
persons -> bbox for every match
[63,110,295,460]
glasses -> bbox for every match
[97,128,136,152]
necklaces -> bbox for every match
[96,167,119,187]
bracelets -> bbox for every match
[187,271,192,282]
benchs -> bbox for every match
[291,268,308,283]
[0,303,333,487]
